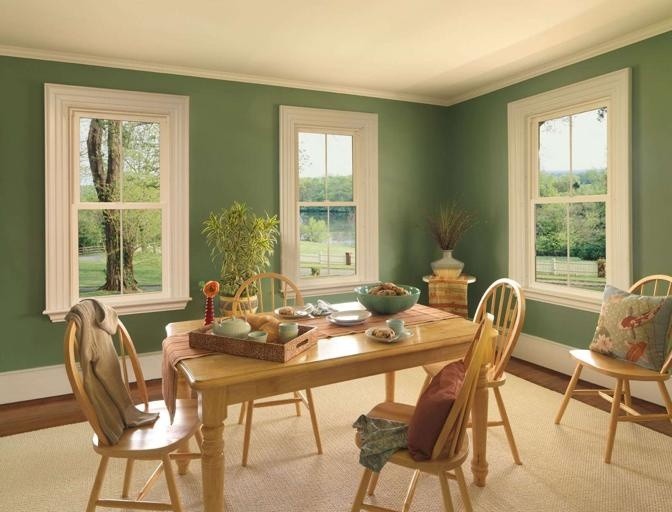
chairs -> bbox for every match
[348,313,496,510]
[552,270,670,466]
[62,296,205,510]
[417,275,527,469]
[230,270,325,467]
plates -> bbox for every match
[273,305,313,319]
[326,310,372,325]
[364,326,413,342]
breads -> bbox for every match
[372,327,395,339]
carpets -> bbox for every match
[2,356,670,512]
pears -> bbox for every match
[368,283,406,296]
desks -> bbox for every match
[160,298,502,512]
[423,273,475,318]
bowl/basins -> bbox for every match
[354,284,420,316]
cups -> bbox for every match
[386,319,405,335]
[247,331,270,344]
[278,322,299,343]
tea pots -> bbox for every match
[210,313,252,339]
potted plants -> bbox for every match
[425,200,485,279]
[200,205,282,317]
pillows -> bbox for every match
[405,357,471,462]
[586,283,671,375]
